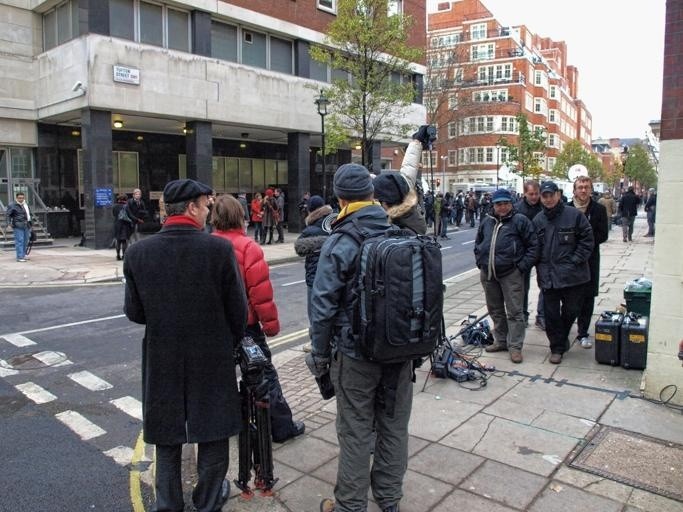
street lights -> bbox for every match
[313,87,331,204]
[493,144,502,193]
[439,154,448,199]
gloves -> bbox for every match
[303,345,335,400]
[414,125,437,150]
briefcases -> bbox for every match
[595,311,623,366]
[620,312,648,370]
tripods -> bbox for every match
[420,238,488,394]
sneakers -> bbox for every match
[274,422,305,442]
[563,338,570,352]
[551,354,561,363]
[276,239,284,243]
[320,499,334,512]
[16,254,29,262]
[384,504,400,512]
[579,337,593,348]
[486,344,507,352]
[511,351,522,362]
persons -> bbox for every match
[420,190,433,229]
[566,176,609,349]
[306,159,413,511]
[296,195,337,353]
[208,195,307,443]
[644,188,656,238]
[618,186,642,242]
[260,190,277,245]
[475,191,492,219]
[113,194,145,260]
[275,188,284,243]
[373,124,440,233]
[474,188,539,362]
[128,189,146,245]
[251,188,262,241]
[122,176,251,512]
[514,181,548,331]
[7,192,31,263]
[511,190,525,203]
[530,182,594,363]
[597,190,616,233]
[430,189,474,240]
[237,191,249,236]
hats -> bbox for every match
[239,190,247,194]
[492,189,511,203]
[540,182,558,194]
[374,172,409,204]
[309,195,325,211]
[163,179,214,203]
[265,189,274,197]
[334,163,375,199]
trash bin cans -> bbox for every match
[624,279,651,318]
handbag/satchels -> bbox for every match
[118,205,132,224]
[30,231,36,241]
[138,215,160,232]
[273,211,280,224]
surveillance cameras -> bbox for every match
[72,80,83,92]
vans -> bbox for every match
[471,187,516,197]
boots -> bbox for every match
[122,248,127,259]
[267,230,273,244]
[116,248,121,260]
[260,230,267,245]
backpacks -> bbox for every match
[333,221,442,363]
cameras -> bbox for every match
[421,125,437,146]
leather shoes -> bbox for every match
[536,318,546,330]
[220,479,229,511]
